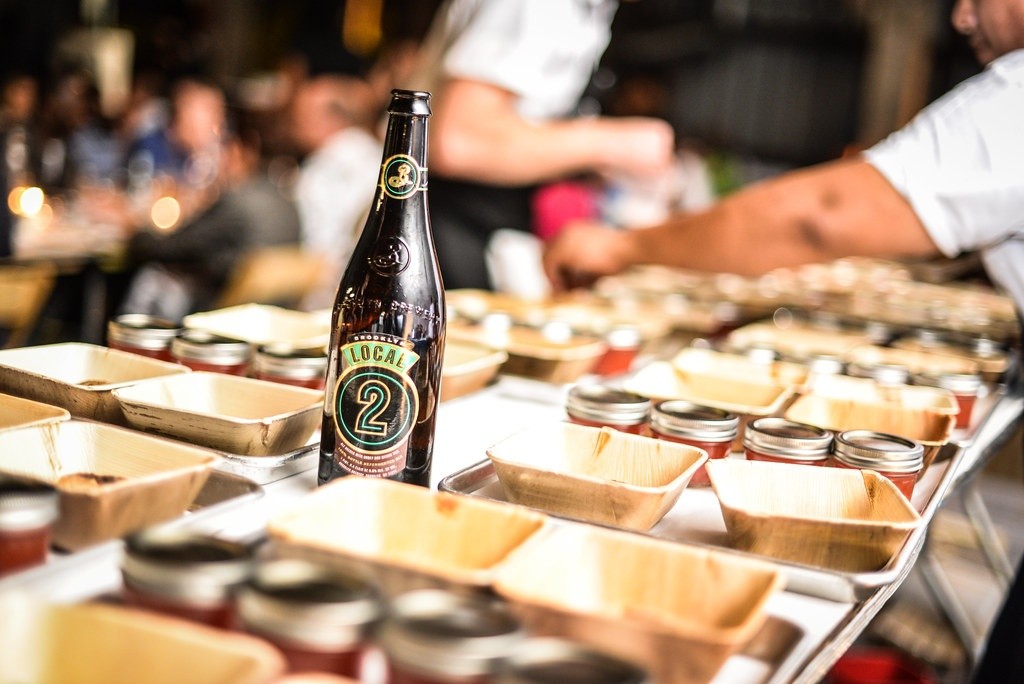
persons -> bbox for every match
[0,52,710,345]
[536,1,1023,683]
[373,0,681,295]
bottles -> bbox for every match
[310,90,449,485]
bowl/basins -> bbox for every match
[0,263,1018,684]
